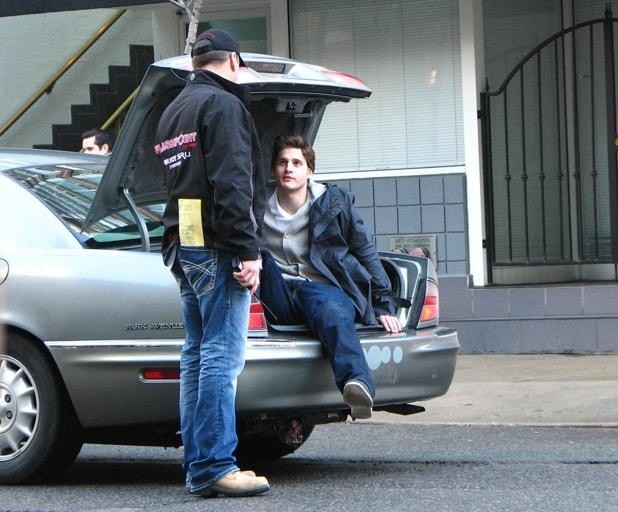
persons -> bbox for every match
[154,26,272,500]
[252,132,404,420]
[79,128,112,156]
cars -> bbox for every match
[2,53,462,497]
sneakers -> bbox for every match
[342,378,373,420]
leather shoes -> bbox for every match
[191,471,270,497]
[185,470,256,500]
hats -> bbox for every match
[191,28,246,69]
